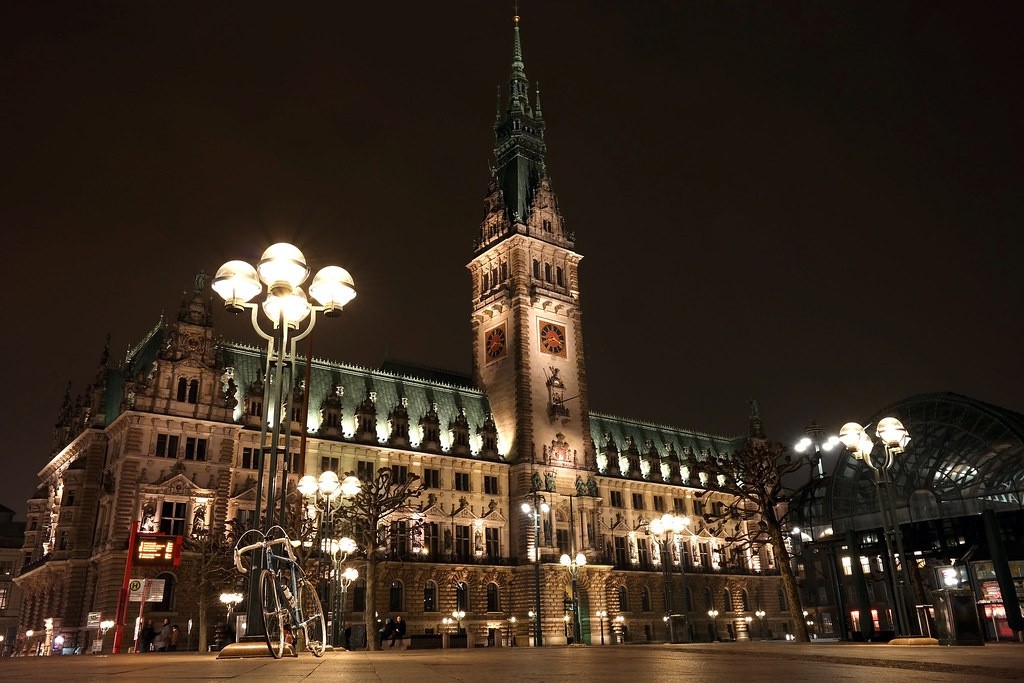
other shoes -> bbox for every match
[389,644,394,647]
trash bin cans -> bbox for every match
[670,615,689,643]
[931,589,985,646]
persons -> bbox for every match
[138,618,180,652]
[379,616,407,648]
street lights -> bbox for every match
[507,616,516,645]
[708,610,720,643]
[528,611,537,647]
[294,470,361,656]
[100,621,114,655]
[840,416,941,647]
[755,611,766,641]
[452,611,465,648]
[220,592,244,643]
[521,488,551,647]
[559,493,589,643]
[595,610,606,645]
[210,242,357,660]
[320,535,360,651]
[792,434,825,479]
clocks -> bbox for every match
[485,328,504,358]
[542,323,565,353]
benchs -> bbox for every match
[381,634,467,649]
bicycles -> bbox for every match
[234,525,327,659]
